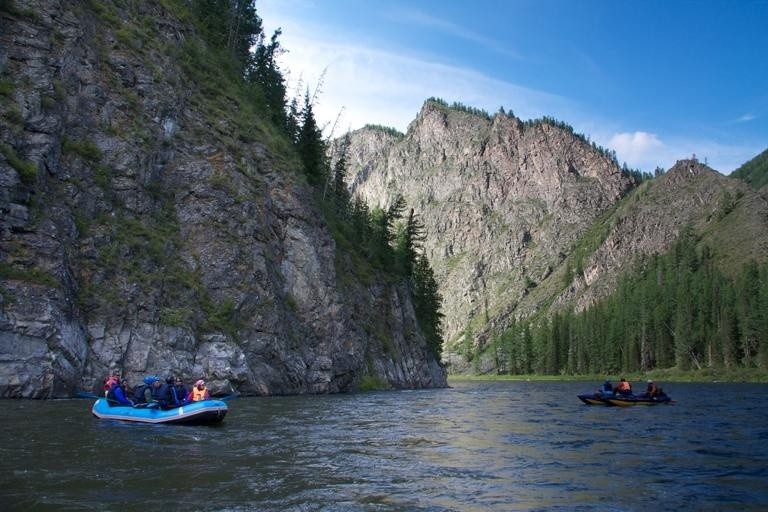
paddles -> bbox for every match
[77,392,120,403]
[220,393,237,401]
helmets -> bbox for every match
[144,375,183,384]
[196,380,205,387]
[605,380,611,384]
[647,380,653,384]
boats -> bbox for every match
[91,397,228,424]
[578,389,670,407]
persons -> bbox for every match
[645,379,659,397]
[103,372,209,409]
[613,378,632,397]
[599,378,613,397]
